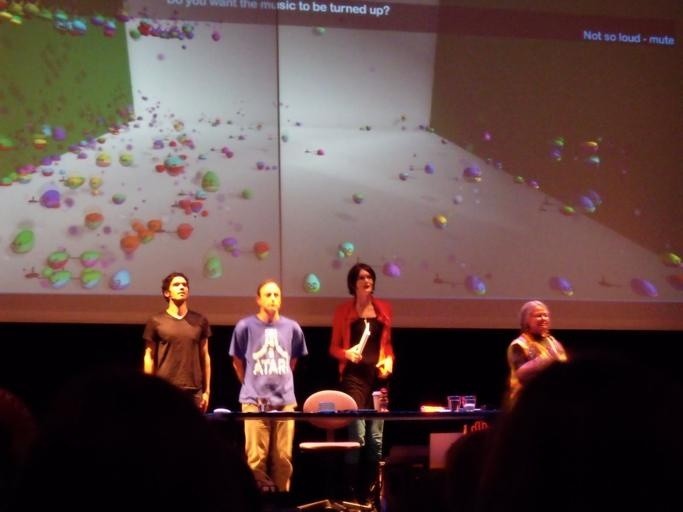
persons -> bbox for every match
[142,266,213,416]
[505,298,570,411]
[2,345,263,511]
[386,345,683,511]
[230,278,311,495]
[327,262,398,504]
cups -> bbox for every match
[372,387,390,412]
[462,396,477,412]
[256,397,271,413]
[447,395,462,413]
[319,400,336,413]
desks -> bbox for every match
[202,410,498,435]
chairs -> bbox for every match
[295,389,373,512]
